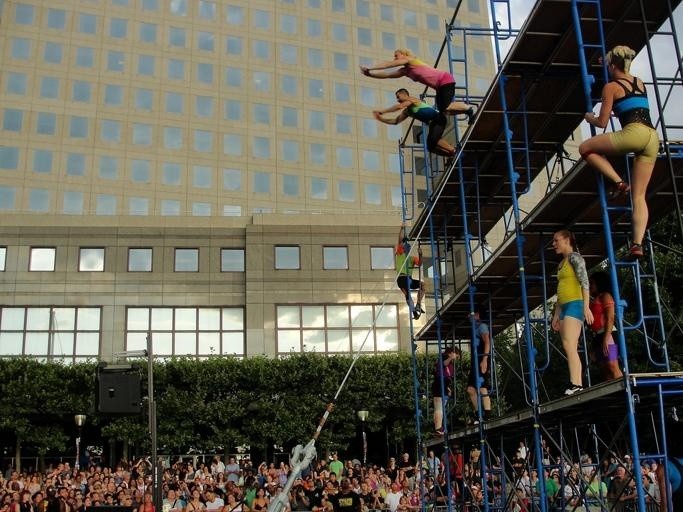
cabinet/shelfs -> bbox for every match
[395,0,683,512]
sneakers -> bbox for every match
[623,245,643,261]
[468,103,478,116]
[608,180,630,200]
[416,305,425,313]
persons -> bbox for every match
[431,346,459,437]
[372,89,457,167]
[589,270,623,379]
[551,229,594,396]
[360,48,478,125]
[0,439,682,511]
[467,310,492,424]
[395,222,427,320]
[579,46,661,261]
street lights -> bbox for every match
[74,414,88,469]
[356,409,368,463]
